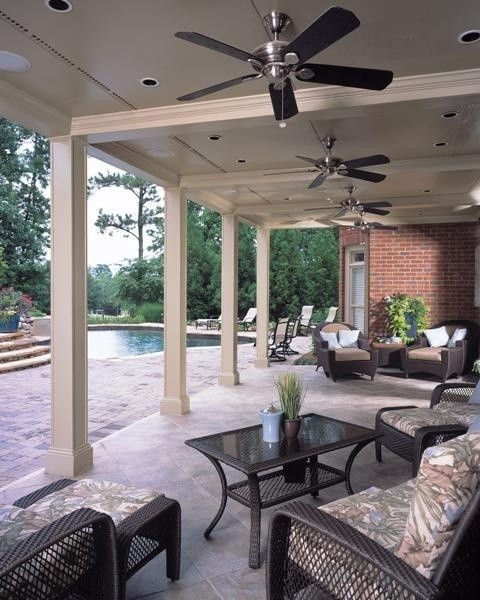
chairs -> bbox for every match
[0,501,119,600]
[194,306,379,382]
[400,319,480,478]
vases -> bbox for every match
[0,314,21,333]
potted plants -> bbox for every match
[271,371,309,440]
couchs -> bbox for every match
[265,430,480,599]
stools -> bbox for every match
[374,406,469,480]
[13,476,182,600]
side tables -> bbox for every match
[371,336,408,379]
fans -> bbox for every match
[293,137,398,236]
[174,5,393,122]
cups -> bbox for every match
[259,402,286,445]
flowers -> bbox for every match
[0,288,33,324]
